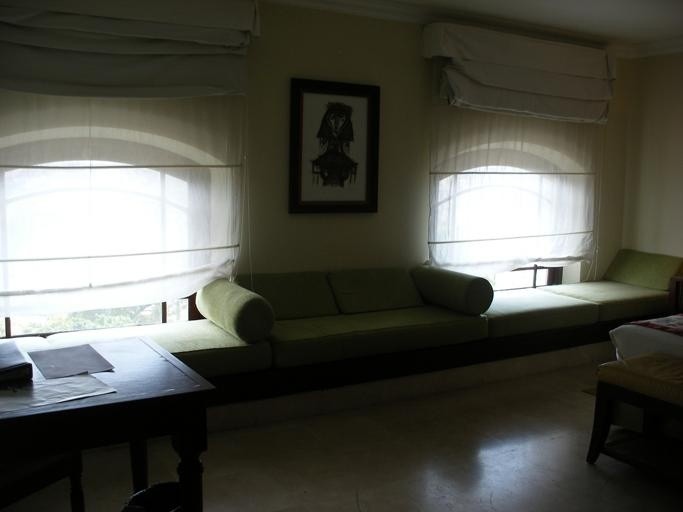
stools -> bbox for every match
[585,349,683,466]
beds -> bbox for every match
[490,247,683,340]
[0,320,261,377]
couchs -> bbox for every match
[195,266,491,371]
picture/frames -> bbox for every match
[287,76,382,215]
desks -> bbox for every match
[0,333,216,512]
